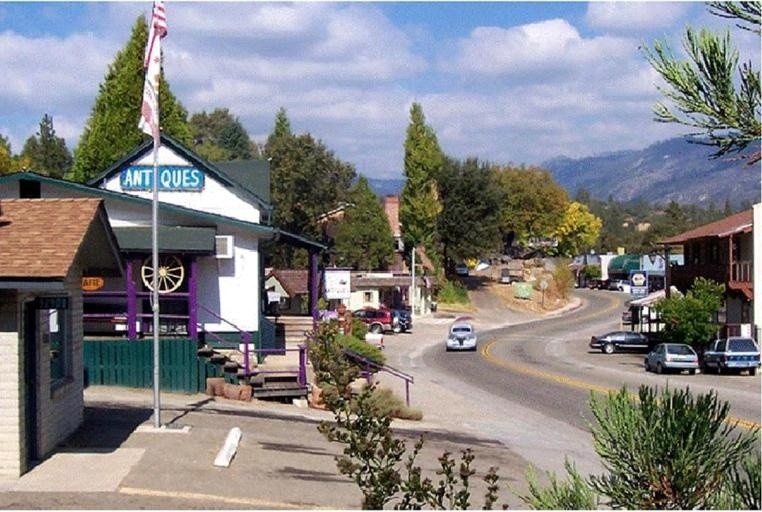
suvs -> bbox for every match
[701,337,759,375]
[339,307,399,334]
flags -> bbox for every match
[138,1,168,148]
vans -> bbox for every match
[454,262,468,275]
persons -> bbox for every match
[628,305,643,332]
[261,283,268,312]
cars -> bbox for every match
[390,308,412,332]
[589,331,651,355]
[643,342,699,376]
[589,279,609,289]
[446,323,478,352]
[608,279,630,292]
